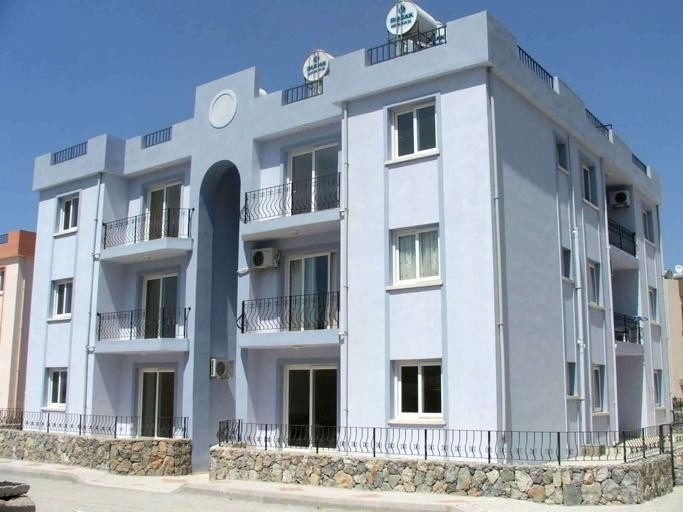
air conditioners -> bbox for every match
[609,190,630,208]
[252,246,280,269]
[211,356,233,381]
